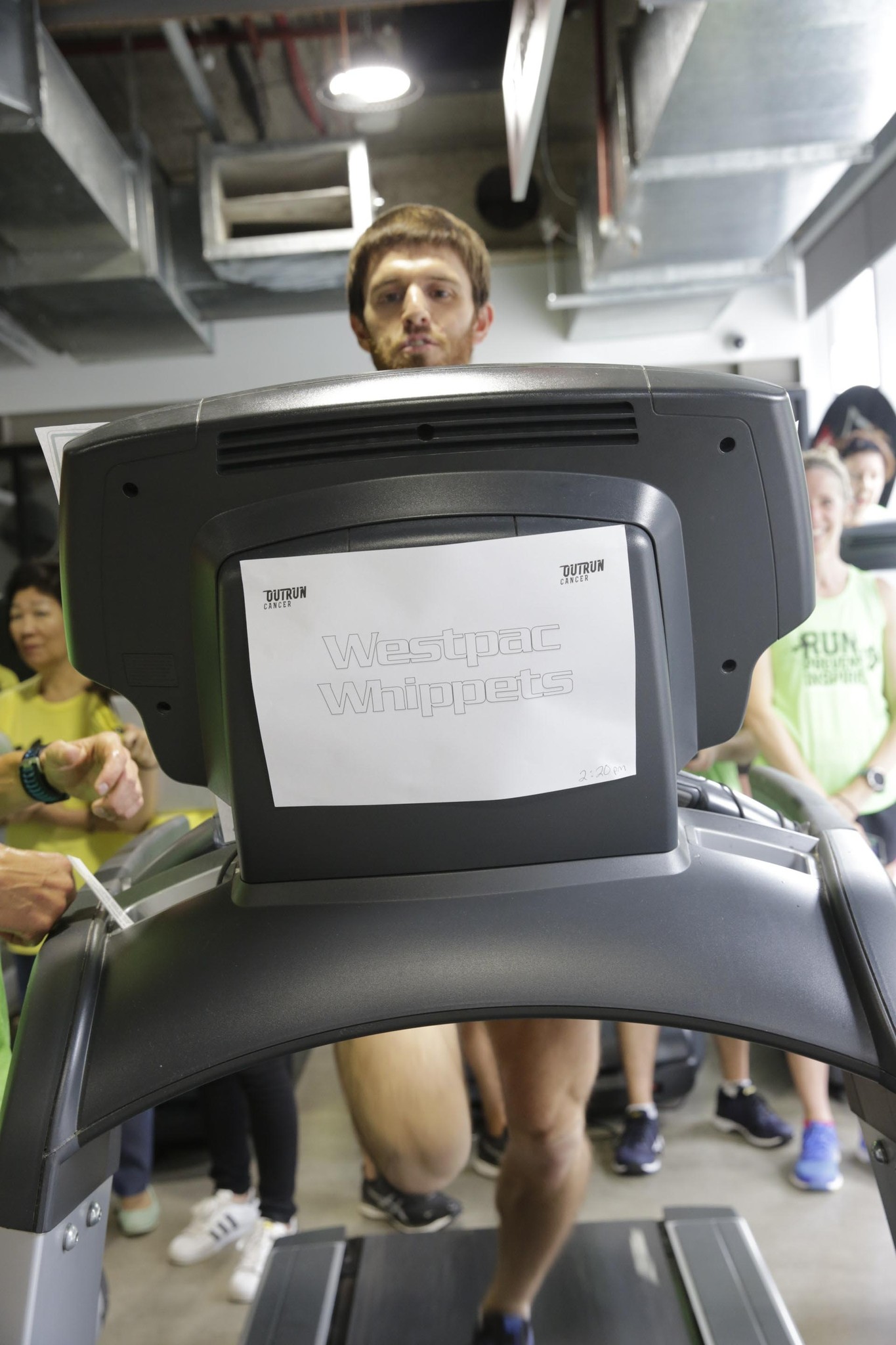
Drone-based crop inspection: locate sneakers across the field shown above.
[168,1189,261,1264]
[359,1173,462,1232]
[473,1130,510,1176]
[229,1219,300,1301]
[612,1110,666,1175]
[790,1124,841,1191]
[714,1083,792,1147]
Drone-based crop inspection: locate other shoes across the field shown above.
[117,1184,163,1237]
[471,1307,535,1345]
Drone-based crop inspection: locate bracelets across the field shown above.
[20,739,69,805]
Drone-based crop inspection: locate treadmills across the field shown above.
[0,356,895,1345]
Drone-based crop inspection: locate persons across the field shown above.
[329,202,604,1345]
[0,434,896,1301]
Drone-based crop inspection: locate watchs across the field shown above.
[859,768,887,798]
